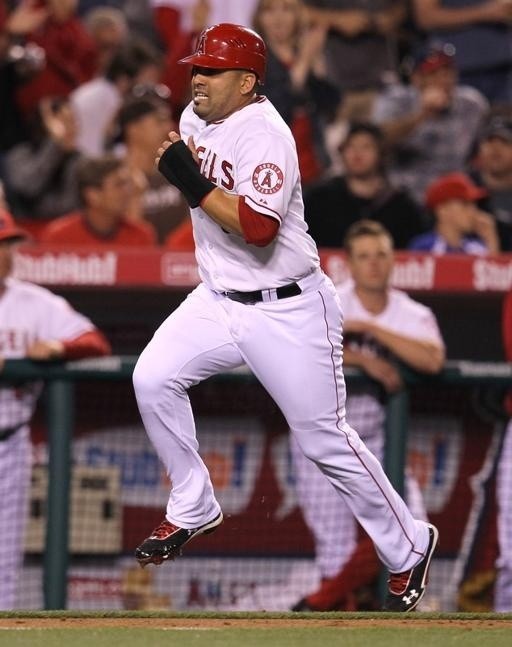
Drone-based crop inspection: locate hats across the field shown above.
[0,212,25,241]
[426,175,488,206]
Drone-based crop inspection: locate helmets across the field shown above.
[177,22,268,81]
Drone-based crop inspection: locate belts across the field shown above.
[220,284,300,304]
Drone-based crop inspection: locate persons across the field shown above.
[297,216,447,606]
[1,215,114,608]
[130,20,440,611]
[491,284,512,608]
[1,1,512,255]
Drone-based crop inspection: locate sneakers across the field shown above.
[381,523,439,612]
[134,509,223,570]
